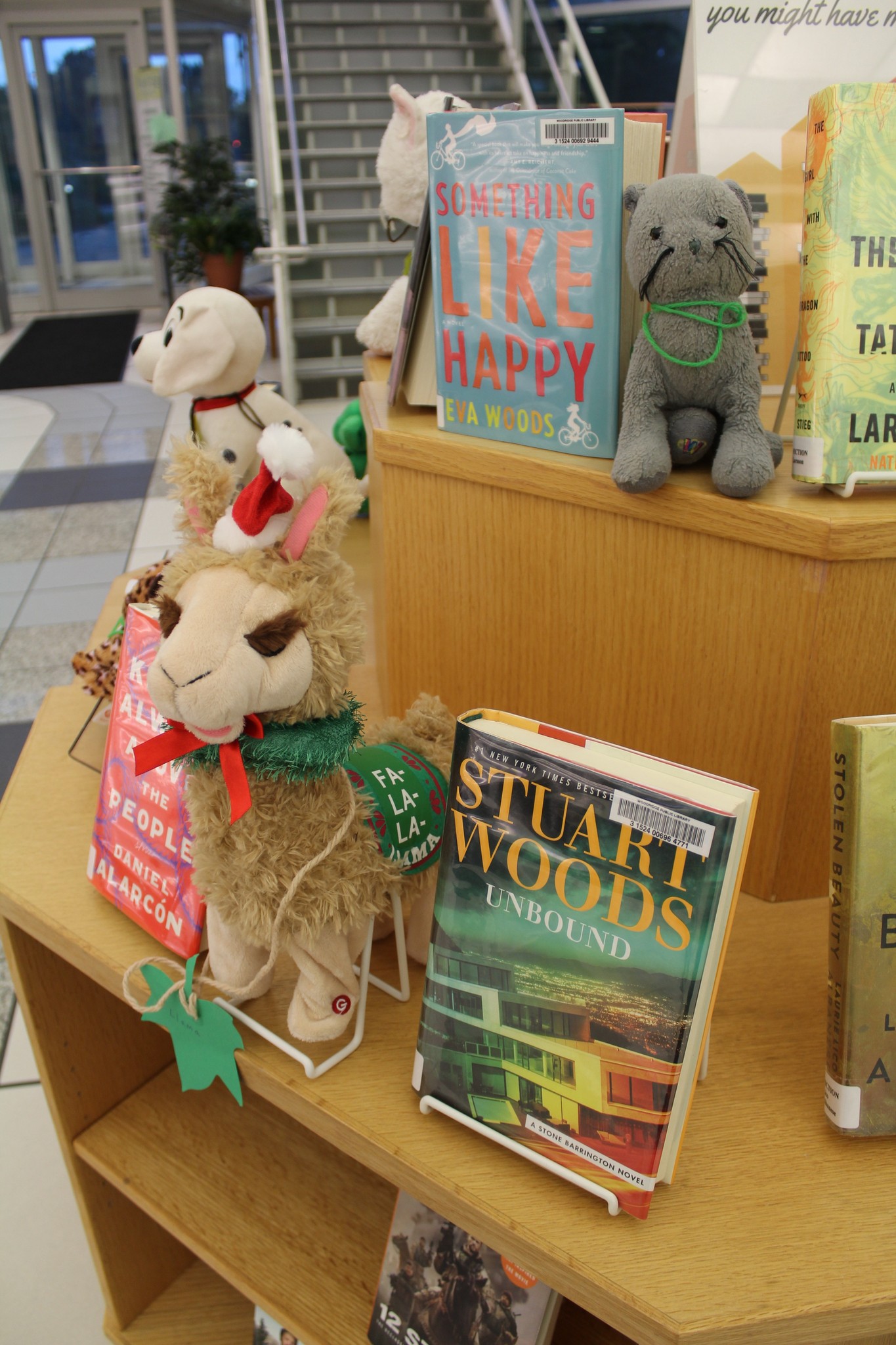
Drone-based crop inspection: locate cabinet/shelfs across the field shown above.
[1,556,896,1345]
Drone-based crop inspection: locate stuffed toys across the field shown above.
[605,169,789,503]
[131,284,378,523]
[134,420,456,1047]
[349,78,479,359]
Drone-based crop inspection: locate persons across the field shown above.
[280,1328,300,1345]
[385,1221,522,1345]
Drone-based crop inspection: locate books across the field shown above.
[822,711,896,1139]
[366,1188,565,1345]
[422,106,672,461]
[789,79,893,488]
[253,1306,304,1345]
[388,183,438,405]
[409,707,760,1222]
[81,598,222,961]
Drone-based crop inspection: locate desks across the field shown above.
[239,289,277,358]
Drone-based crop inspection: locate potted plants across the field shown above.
[147,136,270,293]
